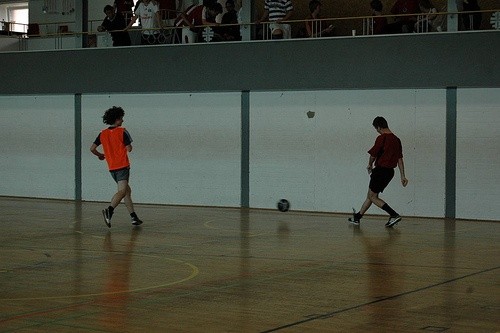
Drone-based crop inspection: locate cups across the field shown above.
[437,26,442,32]
[352,30,355,36]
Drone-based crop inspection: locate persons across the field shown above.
[369,0,389,34]
[97,5,134,45]
[255,0,295,39]
[392,0,423,33]
[462,0,482,32]
[303,0,333,38]
[348,116,408,227]
[221,0,243,41]
[171,3,224,46]
[113,0,135,27]
[421,2,439,19]
[124,0,165,46]
[89,106,144,227]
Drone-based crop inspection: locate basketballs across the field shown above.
[278,199,289,212]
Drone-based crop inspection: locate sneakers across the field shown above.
[132,218,144,225]
[385,214,402,227]
[348,216,360,225]
[102,208,111,227]
